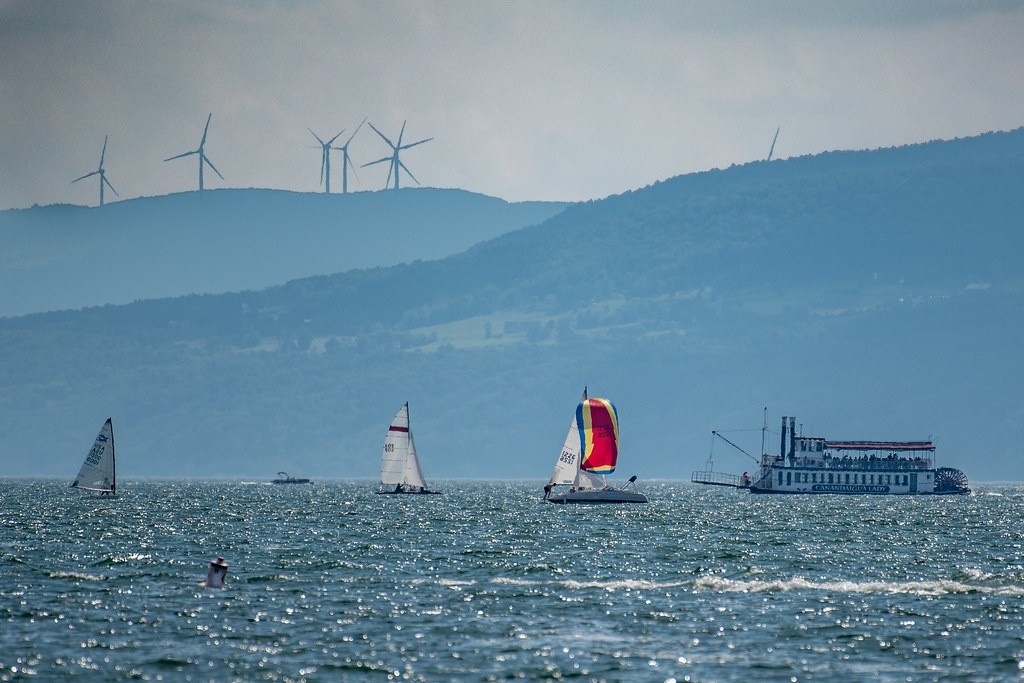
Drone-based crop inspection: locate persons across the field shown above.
[395,483,405,492]
[822,452,923,470]
[743,472,751,487]
[543,483,556,500]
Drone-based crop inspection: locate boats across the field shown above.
[746,415,972,494]
[270,472,313,484]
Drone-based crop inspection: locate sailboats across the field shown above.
[374,401,445,495]
[70,417,124,500]
[542,385,651,503]
